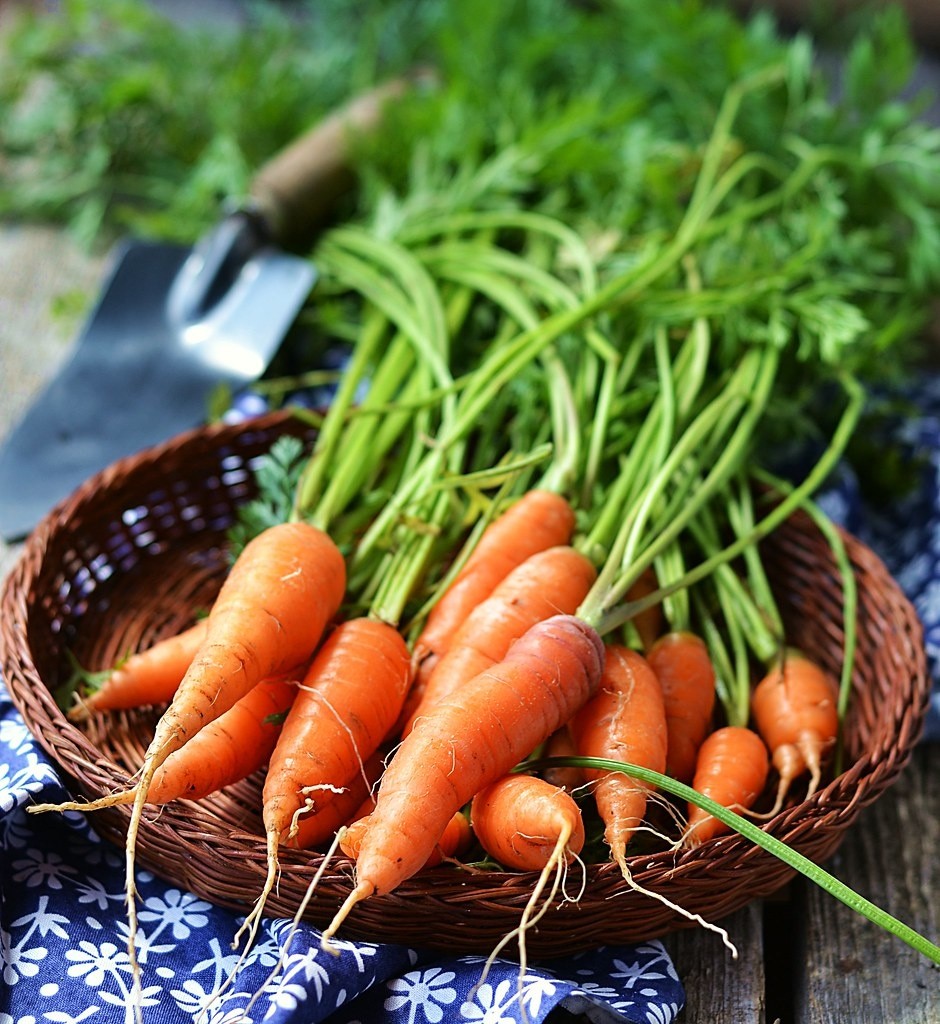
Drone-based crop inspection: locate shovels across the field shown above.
[1,61,442,542]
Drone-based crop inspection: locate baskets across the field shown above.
[0,400,931,961]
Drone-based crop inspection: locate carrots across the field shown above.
[17,490,841,1011]
